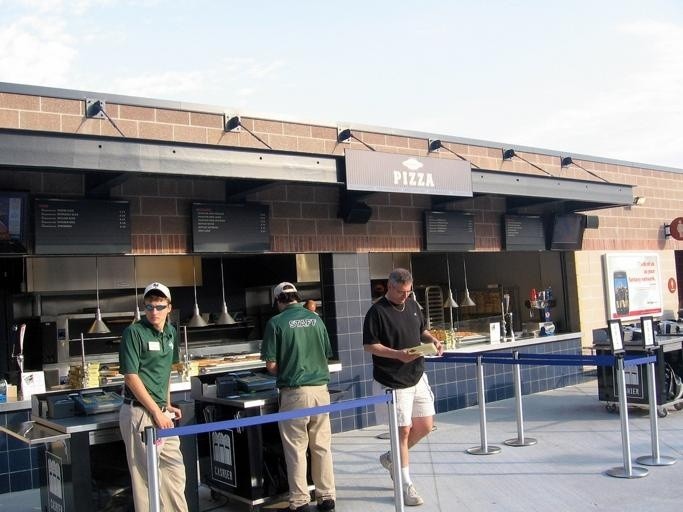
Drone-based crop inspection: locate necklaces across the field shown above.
[384,294,406,312]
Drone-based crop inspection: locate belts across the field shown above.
[122,398,167,414]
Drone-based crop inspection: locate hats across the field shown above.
[142,282,171,301]
[273,282,298,297]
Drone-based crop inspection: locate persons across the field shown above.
[266,282,335,508]
[118,283,189,507]
[362,268,443,504]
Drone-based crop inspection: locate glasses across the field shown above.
[143,303,168,311]
[393,285,414,295]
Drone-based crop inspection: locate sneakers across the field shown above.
[398,490,423,506]
[380,451,393,480]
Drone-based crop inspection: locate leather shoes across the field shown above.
[282,503,311,511]
[318,499,336,511]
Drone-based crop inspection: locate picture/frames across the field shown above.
[602,252,664,321]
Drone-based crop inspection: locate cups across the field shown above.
[0,381,7,404]
[490,322,500,346]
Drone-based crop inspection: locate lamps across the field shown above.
[215,256,237,325]
[459,255,476,307]
[128,255,141,327]
[87,256,111,334]
[186,256,209,328]
[443,253,460,308]
